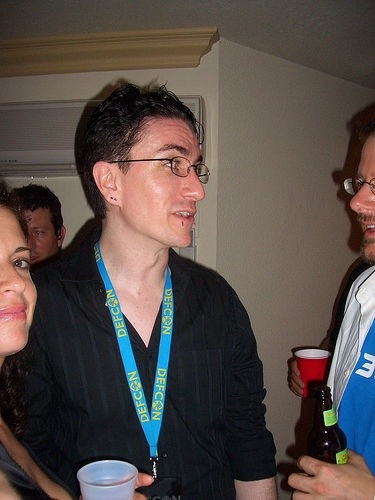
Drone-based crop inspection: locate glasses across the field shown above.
[344,177,375,196]
[111,156,211,185]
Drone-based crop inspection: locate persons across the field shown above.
[0,186,155,500]
[11,183,67,266]
[28,83,278,500]
[287,124,375,500]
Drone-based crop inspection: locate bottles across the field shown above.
[308,386,348,465]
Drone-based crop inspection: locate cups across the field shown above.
[294,349,330,397]
[77,460,138,500]
